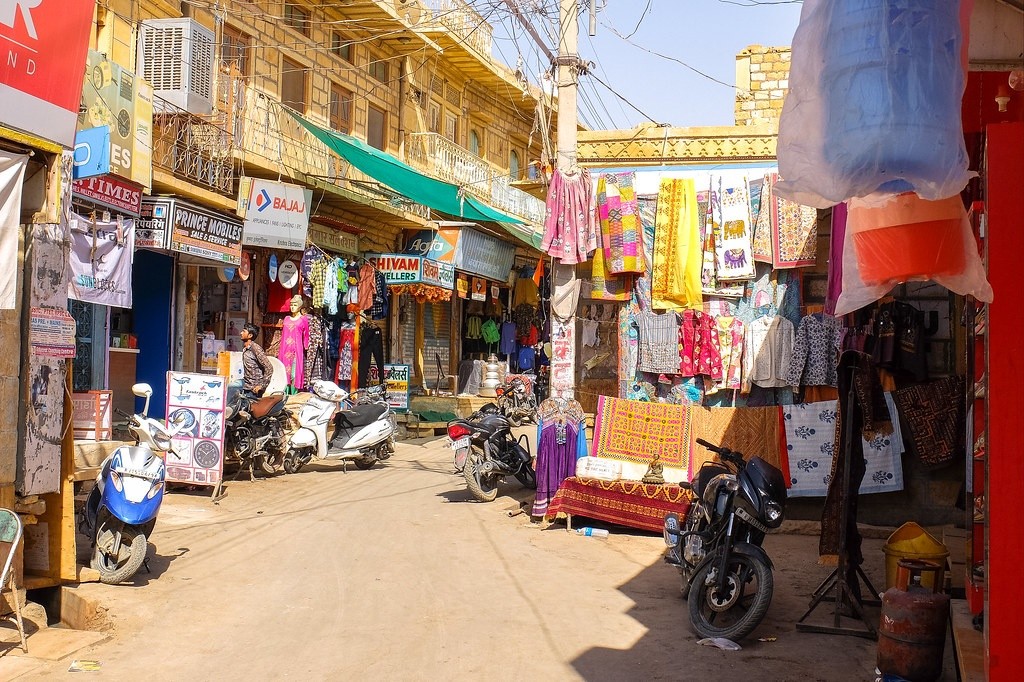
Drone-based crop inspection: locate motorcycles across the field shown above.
[223,390,289,483]
[496,369,539,427]
[447,385,537,502]
[663,438,788,642]
[78,383,186,586]
[284,380,395,474]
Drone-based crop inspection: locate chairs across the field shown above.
[0,508,28,654]
[435,353,457,396]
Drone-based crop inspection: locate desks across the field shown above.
[540,476,692,533]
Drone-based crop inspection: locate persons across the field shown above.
[281,294,309,394]
[241,323,273,398]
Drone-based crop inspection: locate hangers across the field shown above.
[554,388,569,425]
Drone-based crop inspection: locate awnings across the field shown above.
[270,98,527,224]
[496,222,548,255]
